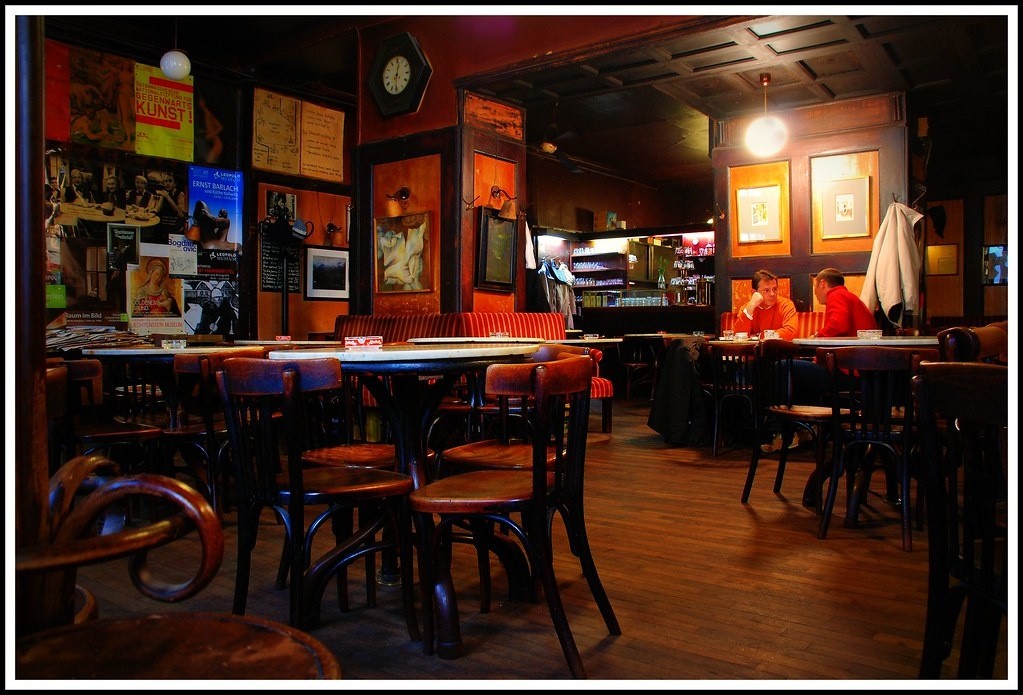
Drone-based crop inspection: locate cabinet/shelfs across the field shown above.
[570,251,625,289]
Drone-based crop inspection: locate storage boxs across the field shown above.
[593,209,618,231]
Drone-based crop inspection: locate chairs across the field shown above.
[17,337,1006,681]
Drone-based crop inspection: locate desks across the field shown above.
[793,336,940,526]
[233,340,342,449]
[80,345,265,496]
[267,344,539,645]
[407,337,545,444]
[544,338,624,448]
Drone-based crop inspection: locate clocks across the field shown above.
[367,30,432,121]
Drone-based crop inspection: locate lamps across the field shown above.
[175,215,202,244]
[744,73,788,159]
[491,186,518,220]
[160,15,191,80]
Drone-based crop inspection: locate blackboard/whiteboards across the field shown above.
[260,233,302,294]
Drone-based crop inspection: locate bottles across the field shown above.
[573,262,599,270]
[573,278,595,286]
[698,274,706,304]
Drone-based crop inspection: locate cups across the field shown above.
[764,330,775,339]
[616,297,668,306]
[723,330,734,339]
[736,333,747,338]
[596,279,623,285]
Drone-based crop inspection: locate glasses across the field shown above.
[757,288,779,294]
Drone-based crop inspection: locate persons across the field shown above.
[69,47,136,145]
[50,169,185,243]
[729,270,799,386]
[759,267,879,454]
[198,99,223,163]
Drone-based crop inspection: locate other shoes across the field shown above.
[761,432,798,453]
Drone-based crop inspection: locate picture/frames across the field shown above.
[474,206,520,293]
[924,242,959,276]
[303,244,350,302]
[735,180,785,244]
[818,175,871,240]
[374,209,435,295]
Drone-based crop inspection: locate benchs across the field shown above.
[460,312,613,436]
[721,312,885,378]
[333,313,459,408]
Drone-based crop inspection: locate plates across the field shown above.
[734,339,751,341]
[760,339,782,343]
[719,337,732,341]
